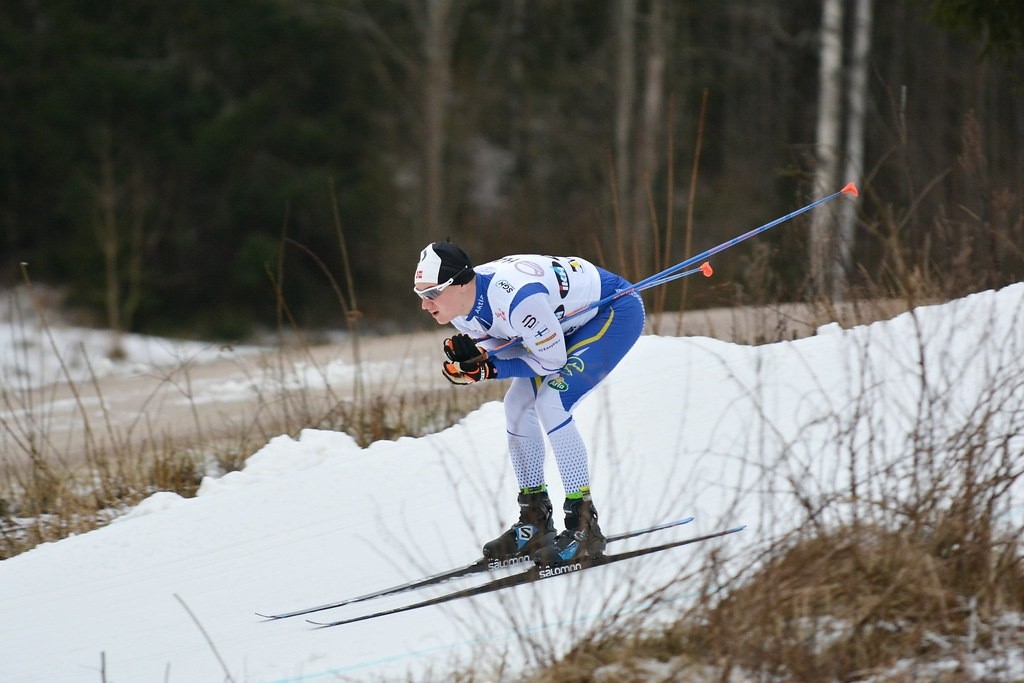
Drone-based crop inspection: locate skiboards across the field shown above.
[253,515,748,628]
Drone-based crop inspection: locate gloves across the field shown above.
[443,333,483,362]
[442,361,497,385]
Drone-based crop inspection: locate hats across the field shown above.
[414,241,475,286]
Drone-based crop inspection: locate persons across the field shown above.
[414,240,645,565]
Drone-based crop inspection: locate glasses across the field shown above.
[414,265,468,300]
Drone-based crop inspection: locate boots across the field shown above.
[483,492,558,560]
[534,498,607,566]
